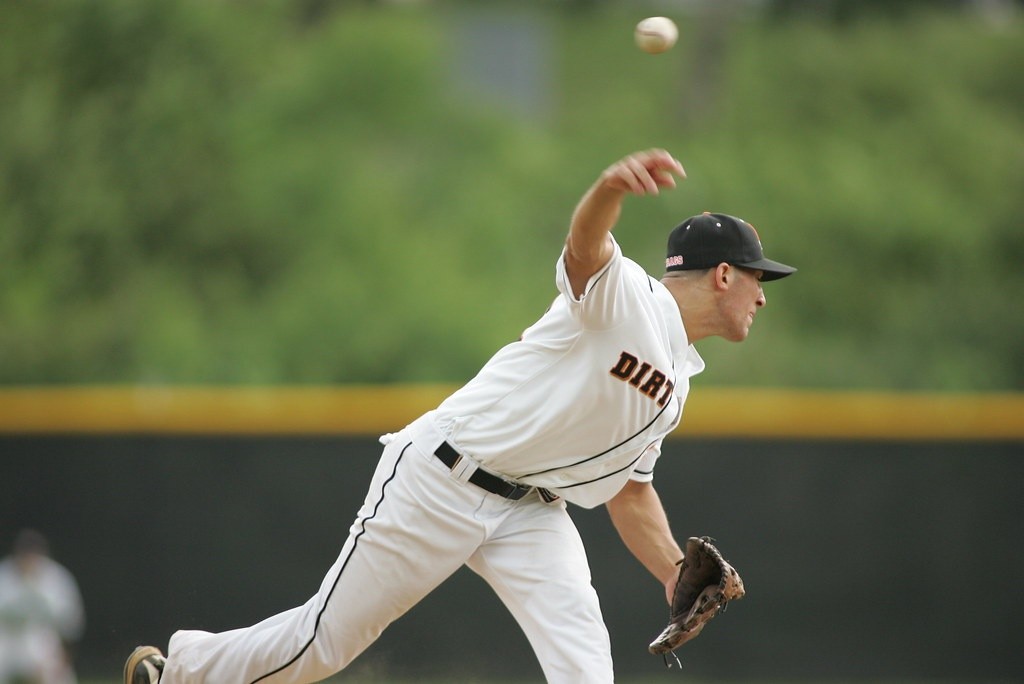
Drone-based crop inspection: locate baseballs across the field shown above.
[630,16,682,54]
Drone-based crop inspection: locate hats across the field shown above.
[665,212,798,282]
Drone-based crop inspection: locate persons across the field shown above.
[122,148,767,684]
[0,526,87,684]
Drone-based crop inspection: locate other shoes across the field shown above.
[123,646,167,684]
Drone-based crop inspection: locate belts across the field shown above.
[435,442,532,500]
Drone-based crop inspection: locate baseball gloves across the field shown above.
[644,533,750,669]
